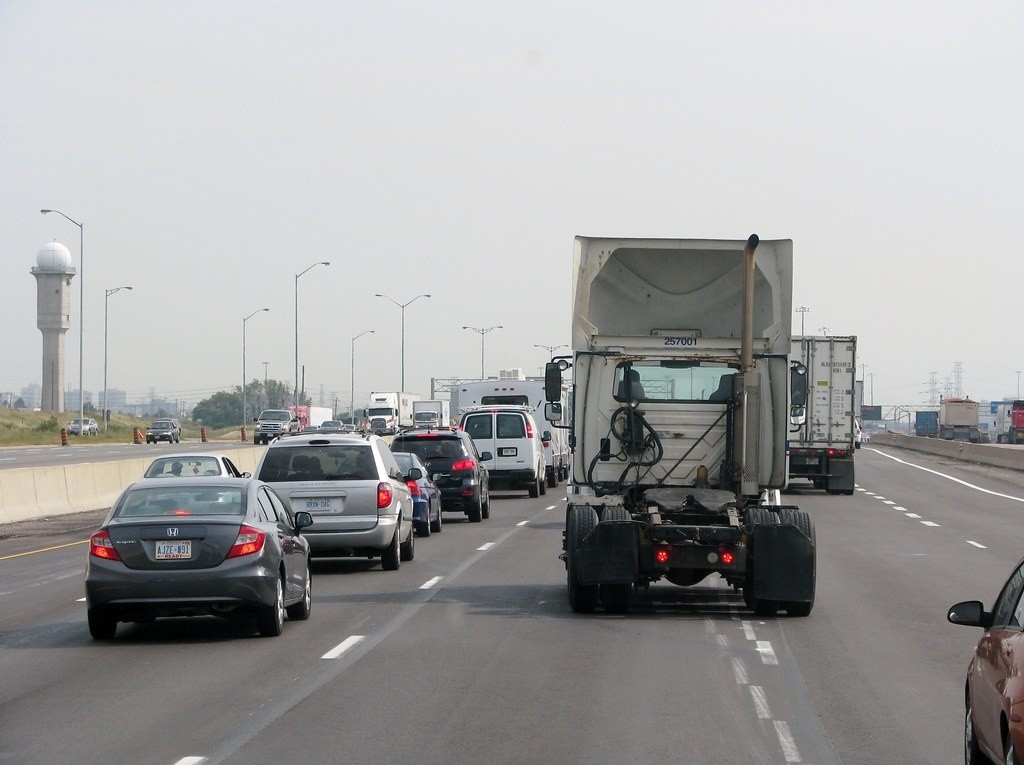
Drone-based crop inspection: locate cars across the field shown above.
[85,405,546,641]
[947,556,1024,765]
[980,432,991,443]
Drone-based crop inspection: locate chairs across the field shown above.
[708,374,736,402]
[620,370,649,398]
[148,498,177,513]
[288,455,312,480]
[137,504,161,516]
[208,503,244,516]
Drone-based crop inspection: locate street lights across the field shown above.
[243,309,270,432]
[859,363,867,405]
[352,331,375,424]
[104,286,133,433]
[41,209,84,438]
[1016,371,1022,400]
[375,294,431,393]
[463,326,504,379]
[868,372,878,406]
[534,344,569,363]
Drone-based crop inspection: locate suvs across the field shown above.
[67,416,98,436]
[855,420,861,449]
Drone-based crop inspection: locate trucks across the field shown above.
[915,411,937,438]
[938,398,979,442]
[993,399,1024,444]
[459,380,571,486]
[364,392,421,435]
[410,400,450,429]
[782,335,857,495]
[543,236,817,617]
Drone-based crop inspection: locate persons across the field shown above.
[172,462,183,476]
[351,452,378,479]
[623,369,640,383]
[289,416,299,431]
[308,456,325,475]
